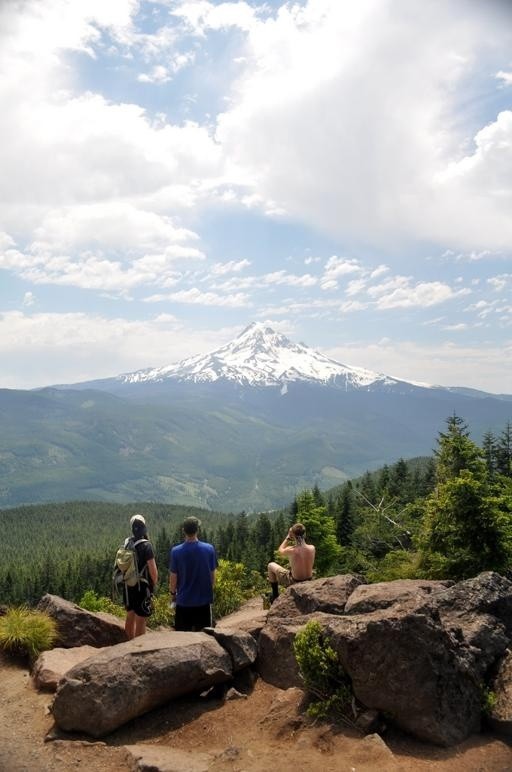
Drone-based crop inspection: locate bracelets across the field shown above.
[287,537,290,541]
[170,592,176,595]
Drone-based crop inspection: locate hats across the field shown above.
[184,516,201,528]
[130,515,145,527]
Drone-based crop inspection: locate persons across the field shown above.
[122,515,159,639]
[169,516,218,631]
[267,523,317,603]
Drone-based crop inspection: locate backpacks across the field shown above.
[112,537,149,587]
